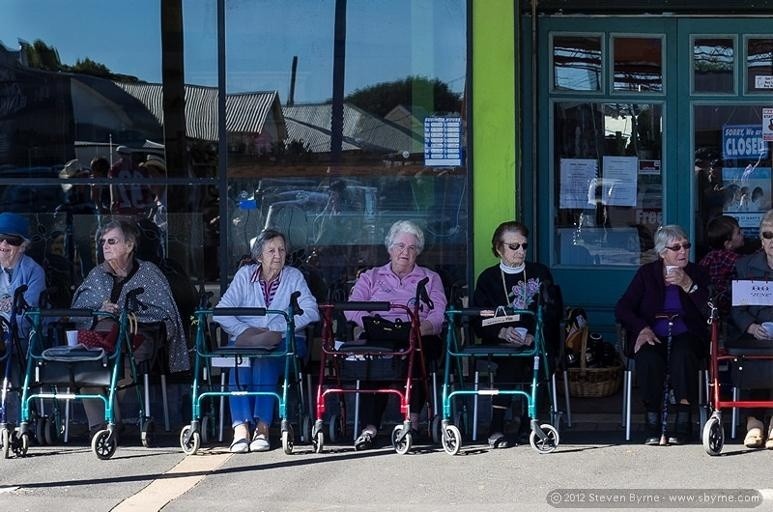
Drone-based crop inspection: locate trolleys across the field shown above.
[306,275,444,458]
[434,278,566,455]
[8,290,159,462]
[178,280,312,456]
[0,283,53,456]
[694,285,772,460]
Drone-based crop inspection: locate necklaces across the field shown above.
[501,270,527,310]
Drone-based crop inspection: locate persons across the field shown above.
[314,181,359,291]
[564,126,589,157]
[471,223,564,448]
[697,216,747,319]
[695,145,771,224]
[725,210,773,449]
[70,218,190,439]
[614,225,716,446]
[343,218,448,450]
[51,141,224,283]
[0,211,52,371]
[213,228,320,454]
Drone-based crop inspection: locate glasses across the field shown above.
[504,243,528,250]
[763,232,773,239]
[665,242,691,251]
[0,234,24,246]
[99,239,118,245]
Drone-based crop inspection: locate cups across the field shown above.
[761,322,773,340]
[666,265,679,276]
[515,327,527,343]
[65,331,77,346]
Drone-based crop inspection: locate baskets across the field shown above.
[556,326,625,398]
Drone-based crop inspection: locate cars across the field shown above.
[204,177,378,239]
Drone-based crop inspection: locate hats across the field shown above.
[139,155,167,172]
[0,212,29,239]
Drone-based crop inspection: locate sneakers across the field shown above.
[487,439,509,447]
[249,439,271,451]
[744,432,763,447]
[230,438,249,452]
[354,433,379,450]
[765,438,773,449]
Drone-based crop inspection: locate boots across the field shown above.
[643,402,661,445]
[668,404,691,444]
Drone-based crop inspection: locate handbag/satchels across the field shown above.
[237,328,282,346]
[361,316,412,349]
[78,324,146,355]
